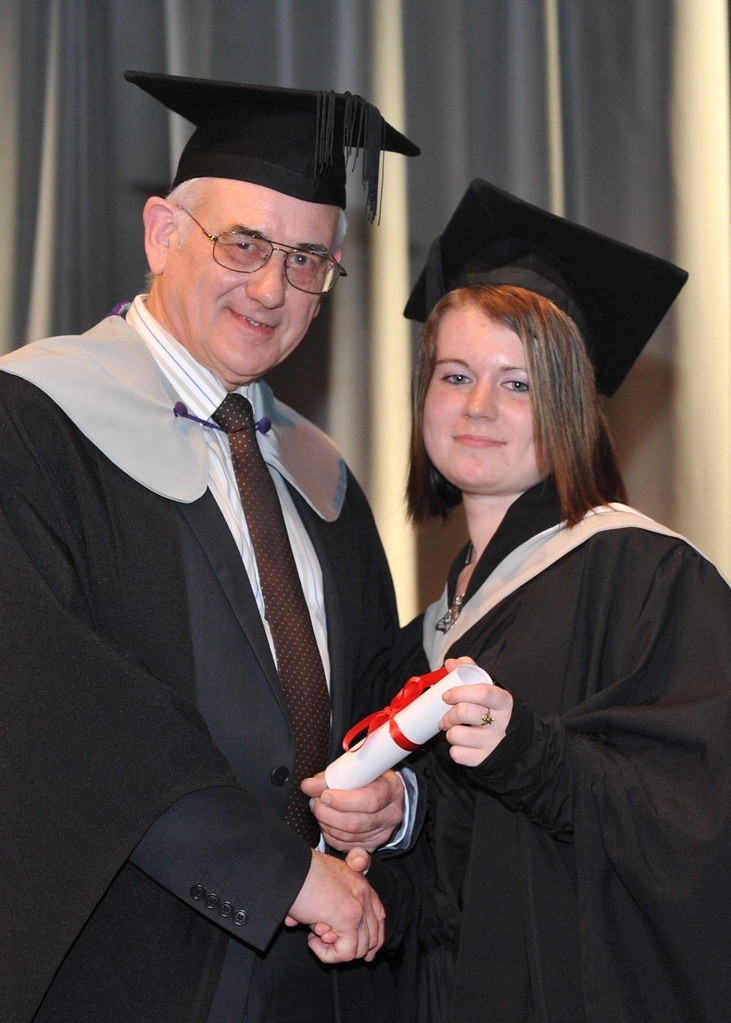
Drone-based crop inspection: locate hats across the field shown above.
[123,65,421,229]
[407,175,688,400]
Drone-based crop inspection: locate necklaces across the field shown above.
[434,542,472,632]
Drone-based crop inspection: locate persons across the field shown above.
[0,69,423,1023]
[285,178,731,1023]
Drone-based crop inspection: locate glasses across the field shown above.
[175,202,347,294]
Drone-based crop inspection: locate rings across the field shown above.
[480,707,494,726]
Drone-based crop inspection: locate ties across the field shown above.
[211,393,331,854]
